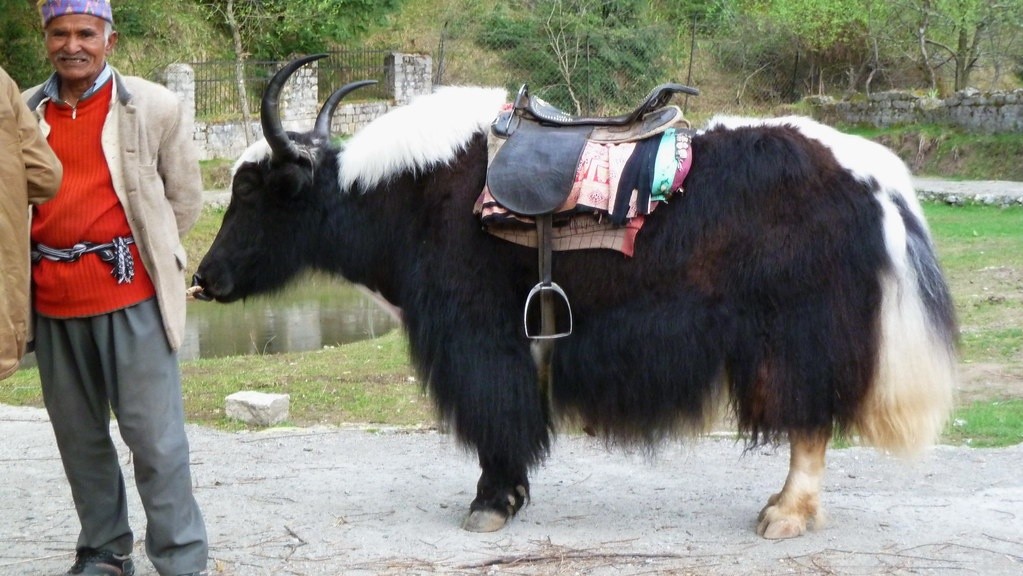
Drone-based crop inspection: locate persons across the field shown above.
[0,65,64,382]
[20,0,209,576]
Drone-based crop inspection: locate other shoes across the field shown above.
[65,548,135,576]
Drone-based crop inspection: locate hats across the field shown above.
[37,0,113,29]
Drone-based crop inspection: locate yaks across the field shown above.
[191,53,962,541]
